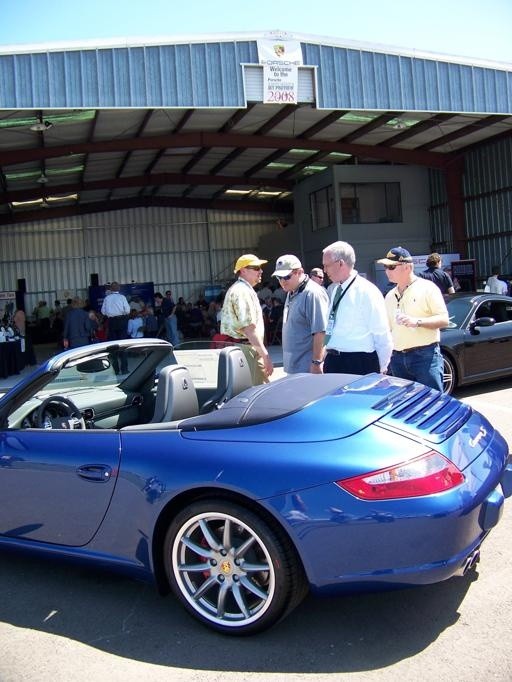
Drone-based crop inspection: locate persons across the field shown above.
[270,255,331,375]
[221,254,273,385]
[14,299,98,367]
[100,268,326,344]
[378,247,449,391]
[419,254,508,296]
[323,242,394,375]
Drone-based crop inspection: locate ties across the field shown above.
[324,285,343,346]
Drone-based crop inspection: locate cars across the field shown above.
[440,292,512,397]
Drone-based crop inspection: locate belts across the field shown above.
[332,349,345,356]
[401,343,436,354]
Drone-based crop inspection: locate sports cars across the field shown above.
[1,337,511,612]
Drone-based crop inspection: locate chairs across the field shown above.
[148,345,252,424]
[269,315,284,345]
[132,326,149,338]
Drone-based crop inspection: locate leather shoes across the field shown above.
[122,370,130,374]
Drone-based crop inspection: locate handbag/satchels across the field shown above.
[211,334,240,348]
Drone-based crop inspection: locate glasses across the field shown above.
[384,264,401,271]
[247,266,262,271]
[275,271,295,281]
[317,275,324,280]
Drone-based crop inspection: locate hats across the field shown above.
[235,254,269,271]
[377,247,413,266]
[426,253,441,267]
[271,254,302,277]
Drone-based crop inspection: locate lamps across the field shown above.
[36,168,49,183]
[30,112,53,131]
[393,117,406,129]
